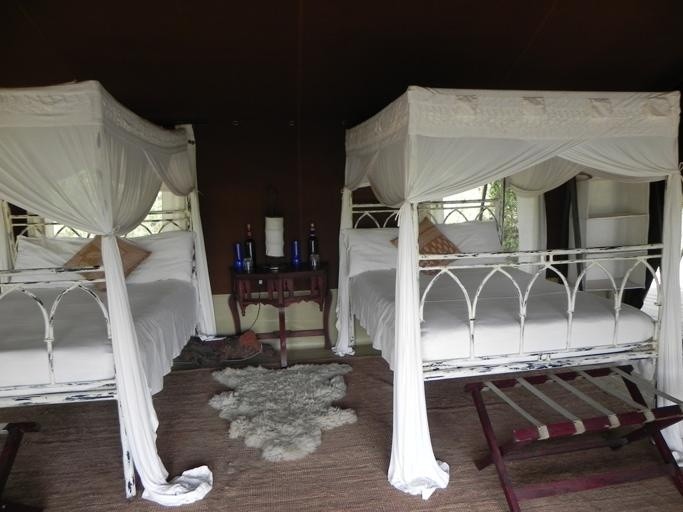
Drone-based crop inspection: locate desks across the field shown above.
[224,262,336,369]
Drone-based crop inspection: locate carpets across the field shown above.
[204,364,359,461]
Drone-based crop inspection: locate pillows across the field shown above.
[54,232,151,289]
[388,217,464,273]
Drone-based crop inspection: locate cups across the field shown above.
[308,253,320,270]
[242,257,254,273]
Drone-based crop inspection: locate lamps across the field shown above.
[261,216,287,271]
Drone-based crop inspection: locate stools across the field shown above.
[457,366,683,510]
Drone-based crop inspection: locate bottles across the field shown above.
[305,223,320,266]
[243,223,256,267]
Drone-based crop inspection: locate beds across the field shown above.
[0,206,218,504]
[336,202,683,449]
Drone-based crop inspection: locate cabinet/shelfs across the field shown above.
[577,173,651,292]
